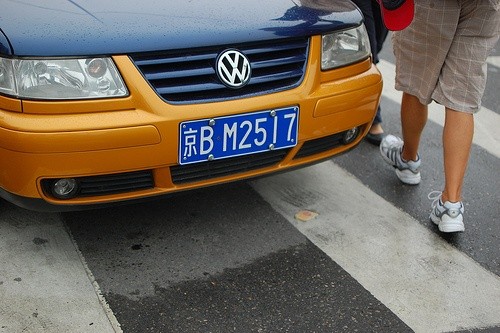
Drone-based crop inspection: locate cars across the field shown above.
[0,0,383,213]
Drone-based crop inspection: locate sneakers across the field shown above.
[427,191,465,233]
[380,135,422,185]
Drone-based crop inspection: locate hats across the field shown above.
[377,0,415,31]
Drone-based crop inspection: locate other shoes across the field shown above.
[365,132,385,145]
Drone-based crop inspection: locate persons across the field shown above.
[380,0,500,233]
[351,0,390,146]
